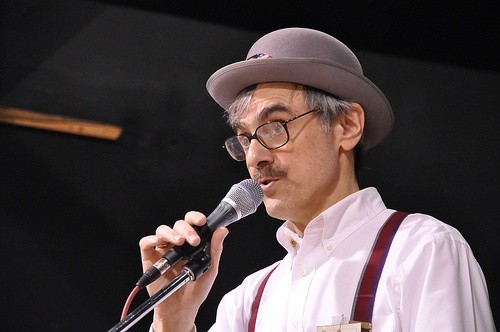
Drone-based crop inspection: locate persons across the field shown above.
[133,25,499,332]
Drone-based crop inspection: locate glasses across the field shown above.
[224,108,314,161]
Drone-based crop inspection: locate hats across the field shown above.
[206,27,395,151]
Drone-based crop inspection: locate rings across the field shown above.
[156,243,169,248]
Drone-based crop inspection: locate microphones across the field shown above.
[136,178,264,290]
